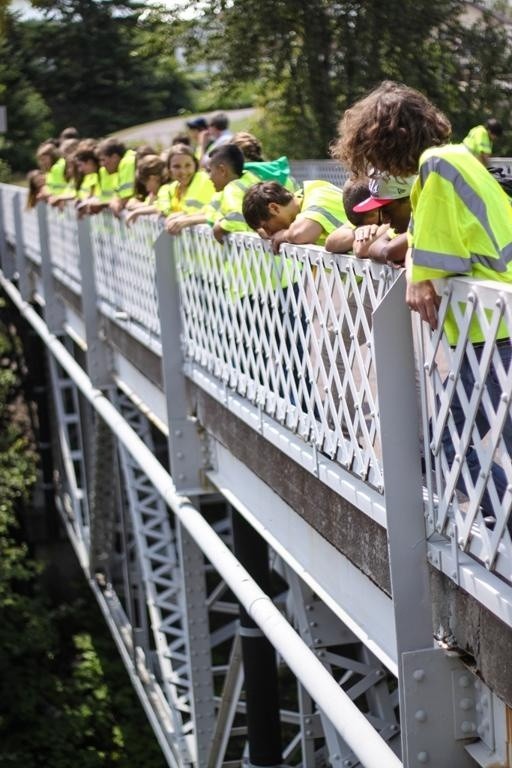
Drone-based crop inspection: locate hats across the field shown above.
[352,167,419,213]
[186,118,207,129]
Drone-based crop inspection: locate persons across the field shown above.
[21,110,298,246]
[321,171,392,255]
[241,177,355,256]
[347,163,421,262]
[457,117,505,172]
[326,78,512,545]
